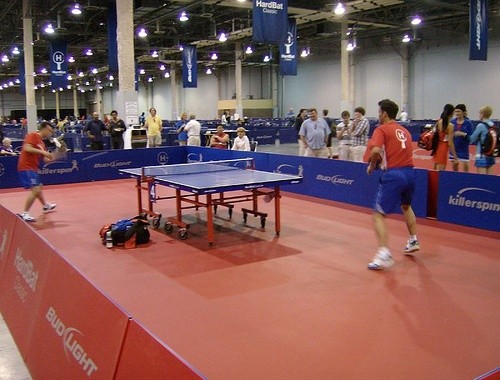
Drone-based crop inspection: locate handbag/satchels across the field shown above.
[100,215,150,246]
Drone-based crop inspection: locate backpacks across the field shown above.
[478,122,500,157]
[418,123,438,151]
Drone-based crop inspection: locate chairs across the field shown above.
[204,133,260,152]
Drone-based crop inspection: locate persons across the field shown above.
[184,114,200,146]
[287,108,295,118]
[144,108,162,147]
[470,105,495,174]
[295,109,307,155]
[176,112,189,146]
[399,111,408,122]
[210,126,229,149]
[299,109,331,158]
[231,127,250,151]
[347,107,369,161]
[363,99,420,269]
[139,112,145,126]
[432,105,459,171]
[336,111,353,160]
[18,122,57,222]
[322,109,333,158]
[222,111,247,129]
[448,104,474,172]
[0,110,127,156]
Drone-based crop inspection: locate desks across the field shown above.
[118,157,304,249]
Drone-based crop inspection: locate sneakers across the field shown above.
[22,213,34,221]
[368,253,395,270]
[401,239,420,253]
[44,203,57,212]
